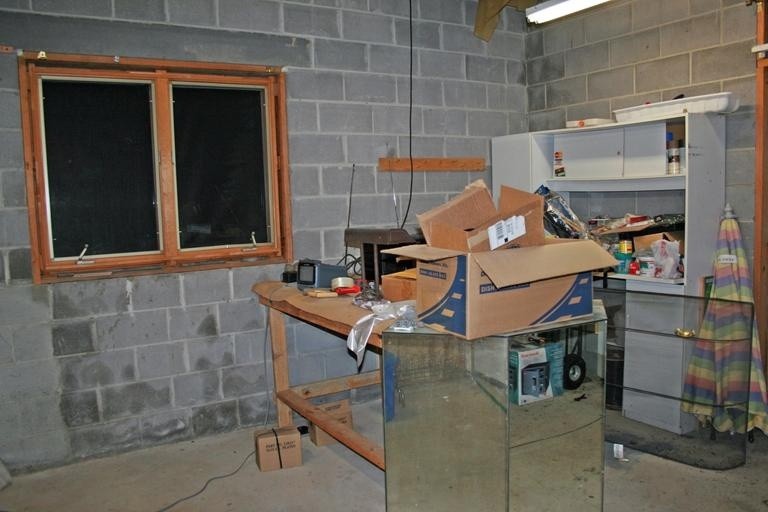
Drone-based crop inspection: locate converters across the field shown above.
[283,270,297,283]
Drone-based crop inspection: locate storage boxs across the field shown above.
[380,240,621,341]
[255,427,302,472]
[415,184,543,253]
[308,398,352,448]
[510,341,564,405]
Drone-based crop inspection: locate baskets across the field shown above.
[566,118,615,128]
[613,92,733,123]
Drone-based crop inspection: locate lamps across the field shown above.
[526,0,614,25]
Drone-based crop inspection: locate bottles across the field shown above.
[553,151,565,178]
[667,140,681,176]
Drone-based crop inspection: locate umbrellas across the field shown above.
[681,202,767,442]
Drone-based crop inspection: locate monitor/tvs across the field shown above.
[297,259,346,290]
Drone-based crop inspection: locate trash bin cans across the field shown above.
[605,338,624,411]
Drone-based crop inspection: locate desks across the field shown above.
[253,281,398,471]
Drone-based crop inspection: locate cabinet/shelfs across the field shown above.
[467,112,727,435]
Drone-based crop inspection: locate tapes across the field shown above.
[331,276,355,291]
